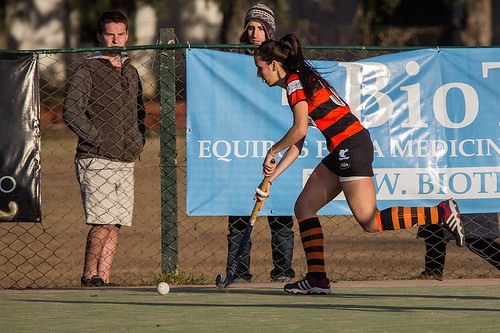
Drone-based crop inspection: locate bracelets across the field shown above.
[268,179,273,186]
[271,145,275,156]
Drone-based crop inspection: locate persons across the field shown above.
[61,9,146,288]
[253,33,464,295]
[216,4,296,283]
[413,213,500,281]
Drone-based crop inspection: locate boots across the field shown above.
[268,228,296,283]
[224,229,253,283]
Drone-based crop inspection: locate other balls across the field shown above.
[157,282,170,296]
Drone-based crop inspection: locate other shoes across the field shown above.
[103,281,120,287]
[80,275,104,287]
[413,270,444,281]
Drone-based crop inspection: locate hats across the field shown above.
[238,2,276,44]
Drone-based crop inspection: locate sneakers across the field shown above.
[437,198,466,247]
[282,273,332,296]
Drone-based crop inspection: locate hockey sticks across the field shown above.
[214,159,276,294]
